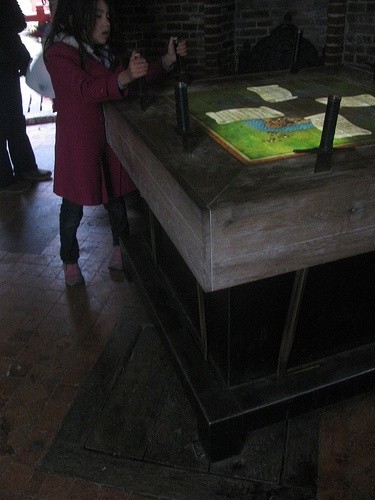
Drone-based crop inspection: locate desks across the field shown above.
[100,60,374,385]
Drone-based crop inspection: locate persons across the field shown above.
[0,0,51,196]
[40,0,188,292]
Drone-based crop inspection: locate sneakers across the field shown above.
[63,263,85,286]
[107,245,124,271]
